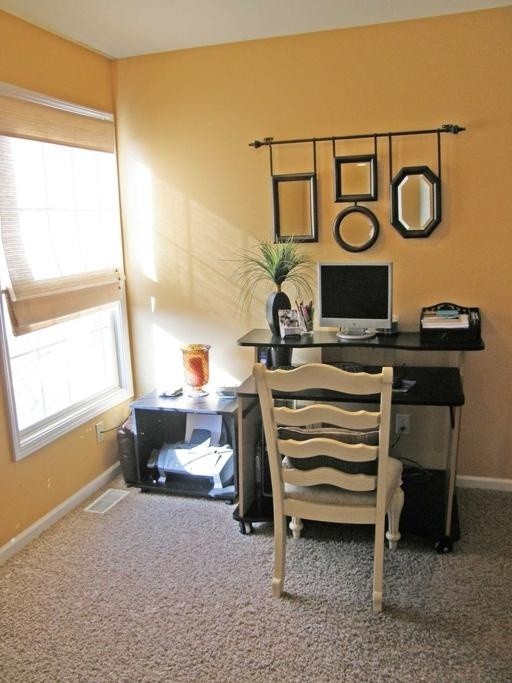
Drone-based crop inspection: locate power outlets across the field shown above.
[96,421,105,444]
[395,414,410,434]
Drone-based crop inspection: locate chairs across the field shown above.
[253,362,404,611]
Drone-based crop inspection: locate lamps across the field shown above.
[179,343,211,397]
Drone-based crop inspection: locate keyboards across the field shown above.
[278,362,366,376]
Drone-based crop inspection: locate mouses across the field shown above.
[393,376,403,389]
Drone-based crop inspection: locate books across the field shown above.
[421,310,469,329]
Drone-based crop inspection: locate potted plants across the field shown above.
[217,232,317,336]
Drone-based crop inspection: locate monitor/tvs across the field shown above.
[317,262,393,340]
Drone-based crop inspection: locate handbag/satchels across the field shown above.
[401,468,461,542]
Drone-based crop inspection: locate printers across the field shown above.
[146,414,235,491]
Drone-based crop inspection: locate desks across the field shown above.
[128,389,239,505]
[234,330,485,554]
